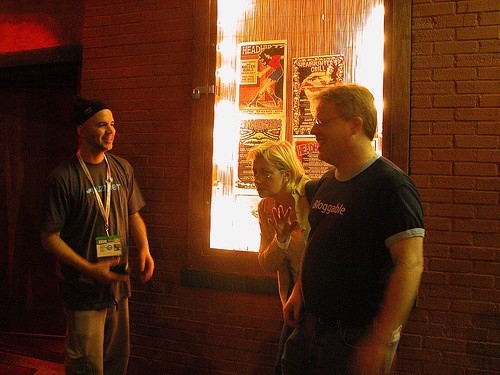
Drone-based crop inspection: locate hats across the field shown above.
[72,96,112,127]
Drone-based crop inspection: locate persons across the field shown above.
[249,141,311,375]
[40,100,154,375]
[116,245,119,250]
[282,84,425,375]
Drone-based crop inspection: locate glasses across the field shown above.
[252,169,288,179]
[313,116,340,126]
